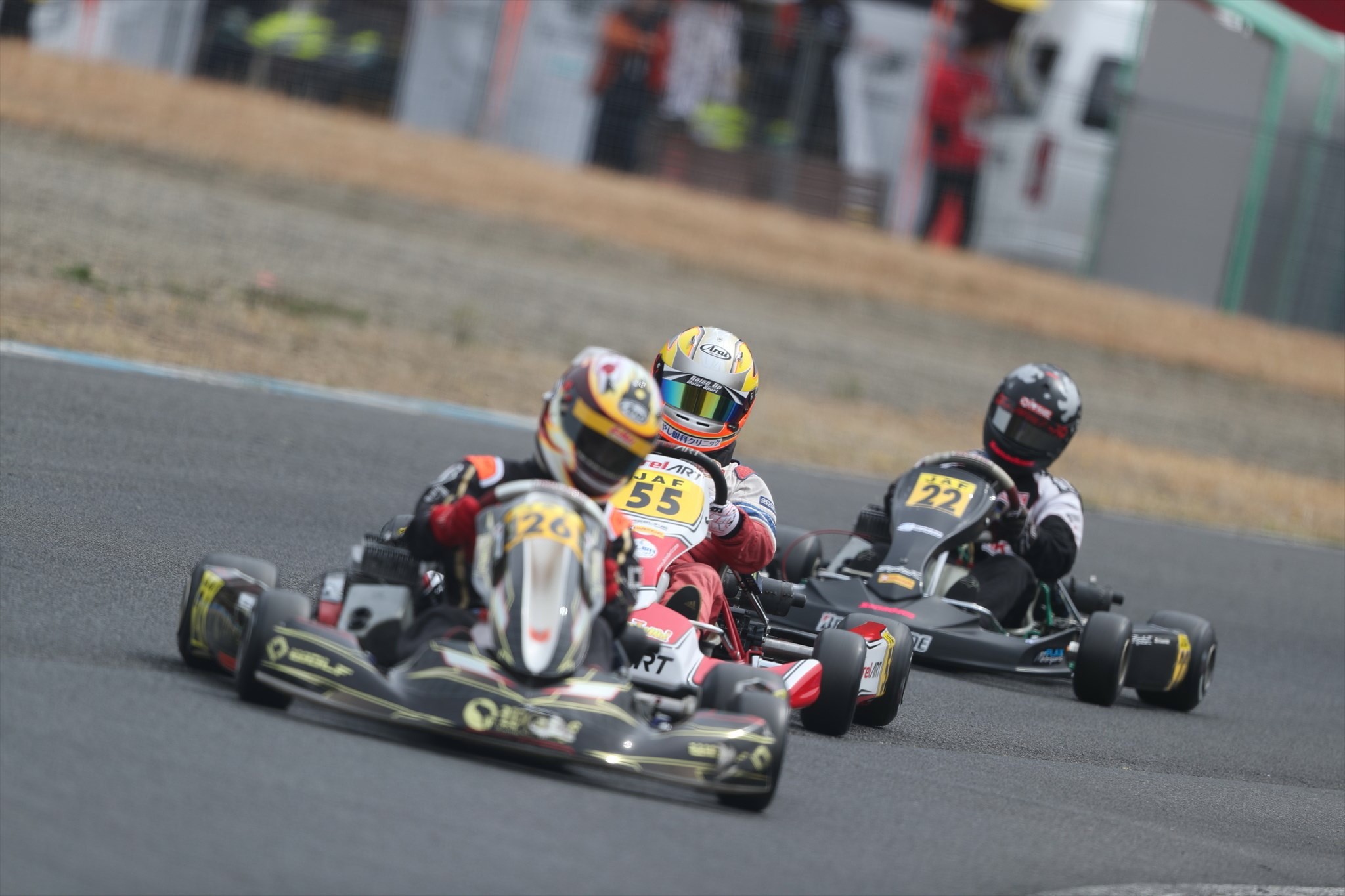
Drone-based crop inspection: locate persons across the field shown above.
[589,0,673,172]
[412,346,664,680]
[837,361,1084,629]
[649,323,778,620]
[733,0,852,202]
[913,29,998,251]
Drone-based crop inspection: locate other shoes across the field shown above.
[663,584,703,621]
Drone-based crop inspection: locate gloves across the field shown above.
[707,499,739,536]
[431,495,480,547]
[1030,516,1077,581]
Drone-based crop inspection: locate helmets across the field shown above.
[533,346,664,507]
[652,326,759,452]
[984,362,1083,473]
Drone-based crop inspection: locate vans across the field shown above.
[963,0,1151,270]
